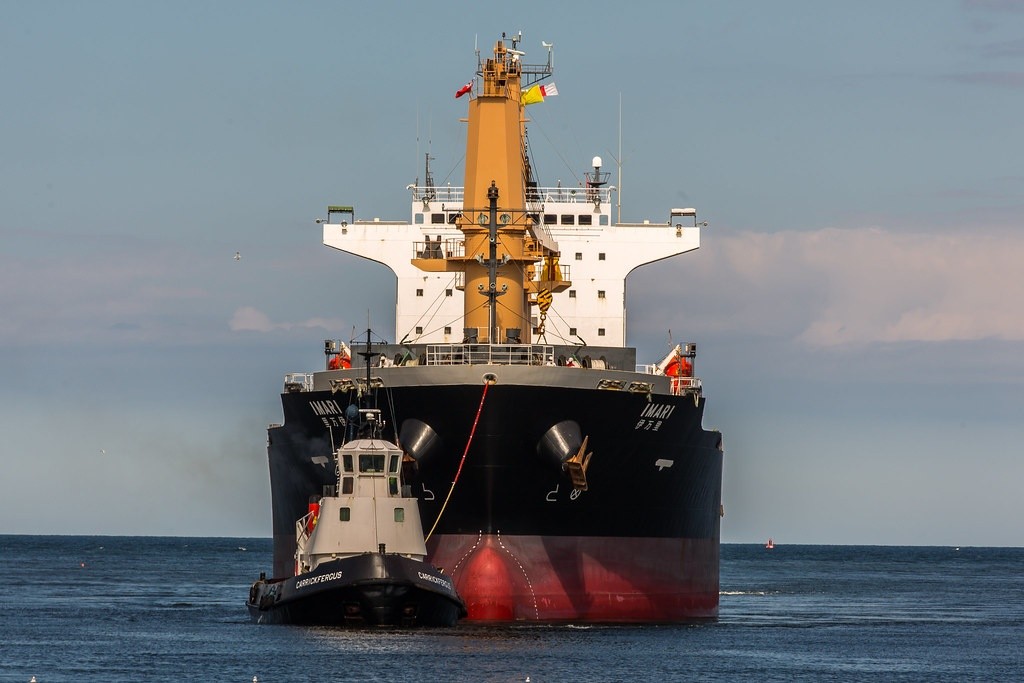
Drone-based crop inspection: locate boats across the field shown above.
[246,306,468,628]
[766,539,775,548]
[265,30,721,626]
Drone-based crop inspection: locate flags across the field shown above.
[455,82,471,99]
[539,85,546,97]
[521,84,543,106]
[544,82,558,96]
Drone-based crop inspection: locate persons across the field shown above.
[546,356,556,367]
[379,356,389,368]
[566,357,580,368]
